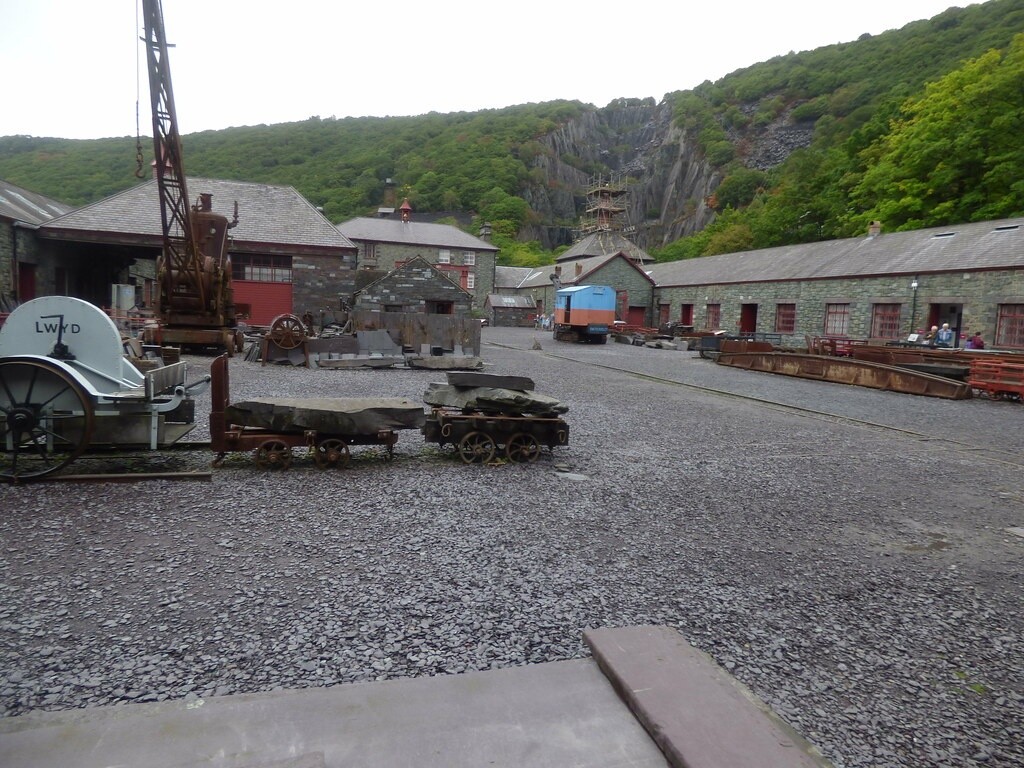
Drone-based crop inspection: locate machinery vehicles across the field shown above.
[133,0,246,356]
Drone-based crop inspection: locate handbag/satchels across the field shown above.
[921,340,930,345]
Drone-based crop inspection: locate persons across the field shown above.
[533,313,556,331]
[923,323,985,350]
